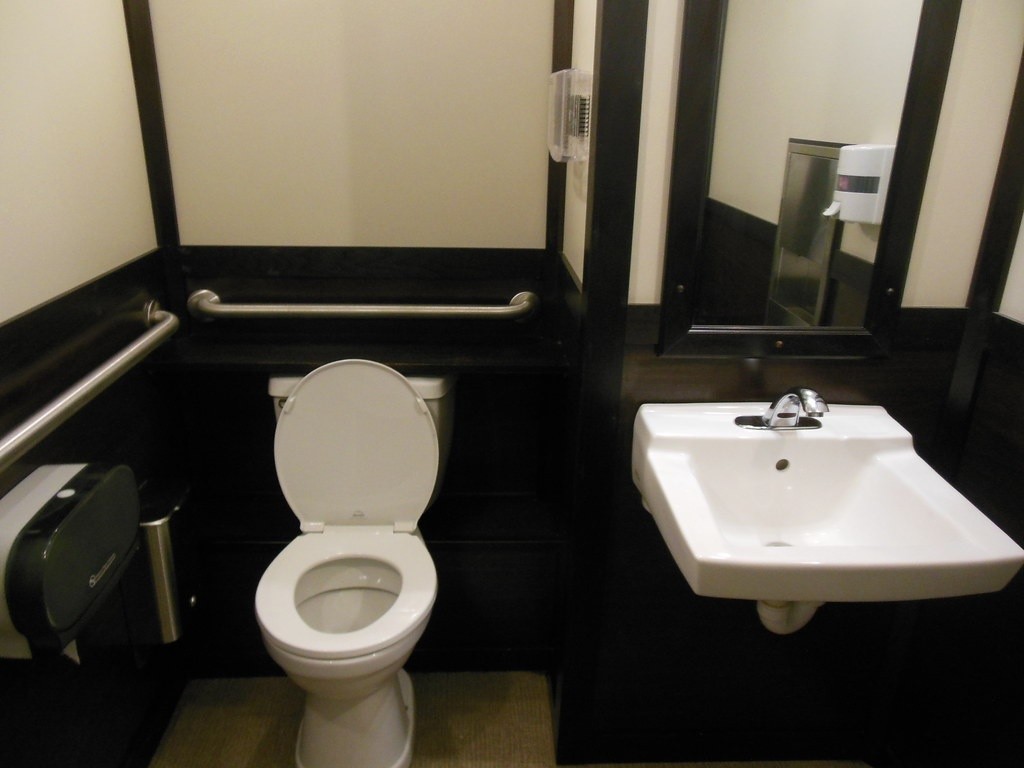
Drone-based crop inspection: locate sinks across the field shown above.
[632,401,1024,602]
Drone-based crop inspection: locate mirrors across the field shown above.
[654,0,962,362]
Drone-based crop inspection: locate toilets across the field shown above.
[254,357,451,765]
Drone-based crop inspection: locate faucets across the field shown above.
[764,386,830,430]
